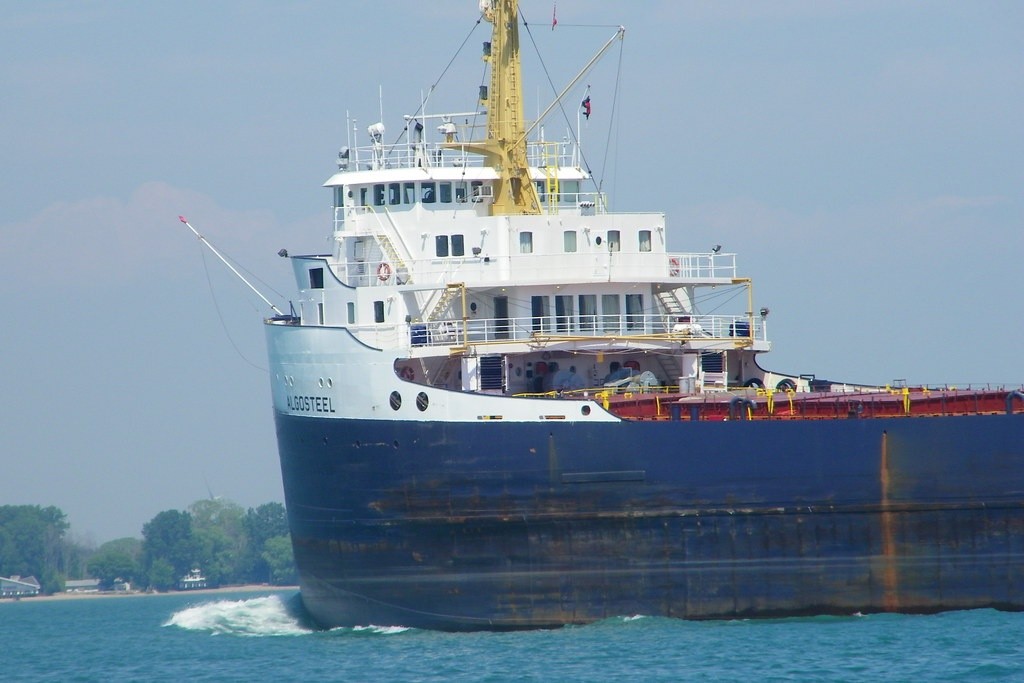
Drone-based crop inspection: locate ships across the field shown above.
[264,105,1023,618]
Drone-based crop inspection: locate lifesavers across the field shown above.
[376,262,390,281]
[400,366,415,381]
[669,257,680,276]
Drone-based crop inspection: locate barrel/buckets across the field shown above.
[688,377,695,393]
[679,377,689,394]
